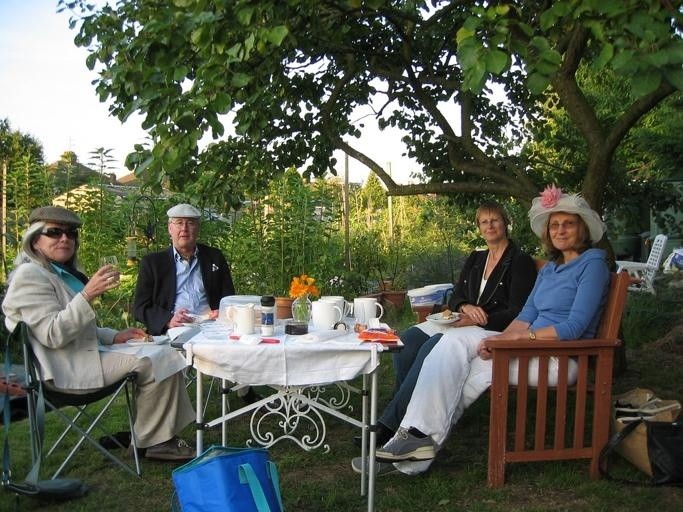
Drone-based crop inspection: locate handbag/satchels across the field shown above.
[597,387,683,486]
[430,288,455,314]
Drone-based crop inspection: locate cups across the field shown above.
[227,301,255,335]
[310,295,385,331]
[99,255,120,291]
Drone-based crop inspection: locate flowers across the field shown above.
[285,272,322,318]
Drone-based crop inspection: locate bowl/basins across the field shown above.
[200,321,233,338]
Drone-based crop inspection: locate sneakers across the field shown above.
[375,427,435,463]
[350,456,401,478]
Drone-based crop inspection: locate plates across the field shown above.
[423,310,463,324]
[124,334,167,348]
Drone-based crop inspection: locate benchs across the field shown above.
[414,270,632,490]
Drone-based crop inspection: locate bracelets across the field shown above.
[80,290,91,301]
[461,303,467,313]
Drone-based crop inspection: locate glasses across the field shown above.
[548,220,578,228]
[38,227,78,240]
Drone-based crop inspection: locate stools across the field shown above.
[172,348,233,417]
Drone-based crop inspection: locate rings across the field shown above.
[473,314,477,318]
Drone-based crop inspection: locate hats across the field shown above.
[166,204,201,219]
[526,182,607,245]
[28,205,83,227]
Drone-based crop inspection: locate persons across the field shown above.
[0,205,195,460]
[133,204,254,403]
[351,212,611,477]
[352,200,537,452]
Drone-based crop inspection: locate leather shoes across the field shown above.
[240,386,255,402]
[350,422,394,448]
[144,434,196,459]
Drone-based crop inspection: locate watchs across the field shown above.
[528,329,536,340]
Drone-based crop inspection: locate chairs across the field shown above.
[614,234,669,296]
[5,318,144,496]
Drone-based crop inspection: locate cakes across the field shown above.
[441,310,454,320]
[143,334,153,342]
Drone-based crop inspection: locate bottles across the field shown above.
[259,295,276,337]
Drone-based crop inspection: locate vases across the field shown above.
[352,280,433,324]
[290,294,313,328]
[273,297,294,306]
[273,306,292,320]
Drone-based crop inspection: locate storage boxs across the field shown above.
[406,282,454,312]
